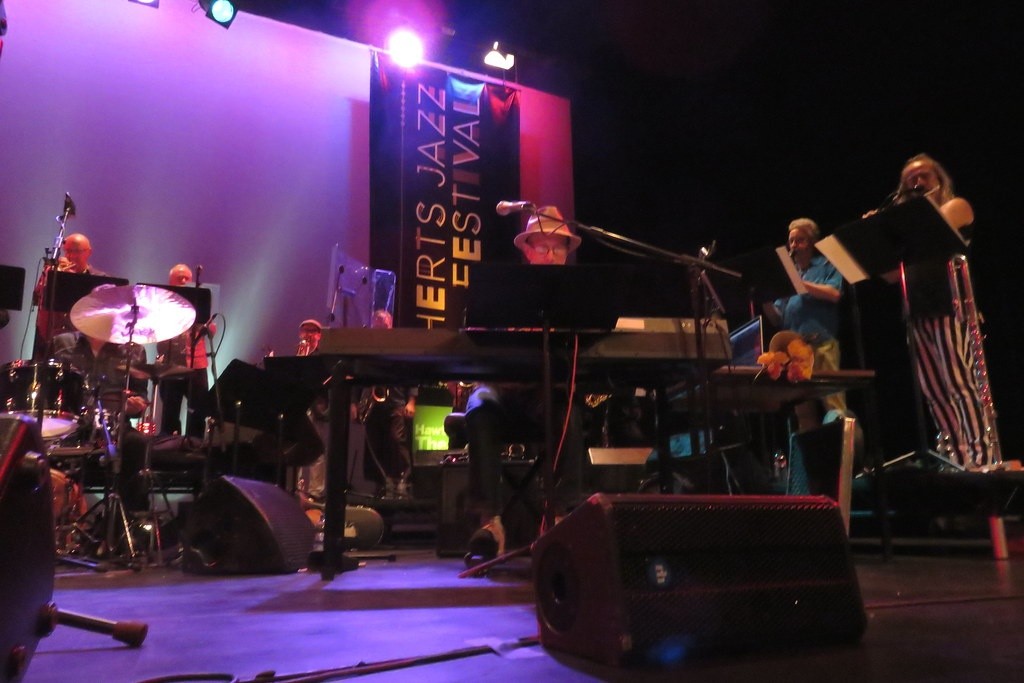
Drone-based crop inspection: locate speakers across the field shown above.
[208,359,325,466]
[436,463,537,551]
[182,475,316,577]
[530,492,867,667]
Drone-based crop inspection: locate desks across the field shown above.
[264,355,729,579]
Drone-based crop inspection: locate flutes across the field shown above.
[948,251,1009,473]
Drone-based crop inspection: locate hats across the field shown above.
[514,206,581,253]
[298,320,322,329]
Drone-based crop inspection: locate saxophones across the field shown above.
[296,335,312,356]
[353,383,390,426]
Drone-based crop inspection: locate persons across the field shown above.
[463,206,597,556]
[759,217,871,472]
[290,310,420,500]
[862,153,1003,474]
[32,233,217,442]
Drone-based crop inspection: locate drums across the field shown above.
[51,466,90,560]
[1,359,91,443]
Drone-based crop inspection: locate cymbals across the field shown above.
[70,285,197,344]
[119,359,194,377]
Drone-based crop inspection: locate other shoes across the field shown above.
[468,516,505,561]
[382,491,414,500]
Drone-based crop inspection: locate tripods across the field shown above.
[75,306,180,572]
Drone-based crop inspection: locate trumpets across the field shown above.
[46,259,77,279]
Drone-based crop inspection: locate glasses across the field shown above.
[62,246,90,254]
[301,327,319,334]
[526,241,569,256]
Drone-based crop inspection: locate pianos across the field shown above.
[317,318,733,570]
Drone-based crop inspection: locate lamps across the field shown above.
[198,0,239,29]
[482,39,518,83]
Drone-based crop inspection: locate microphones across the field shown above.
[496,200,533,216]
[64,192,76,216]
[914,184,924,192]
[195,314,217,345]
[788,248,798,259]
[88,378,102,408]
